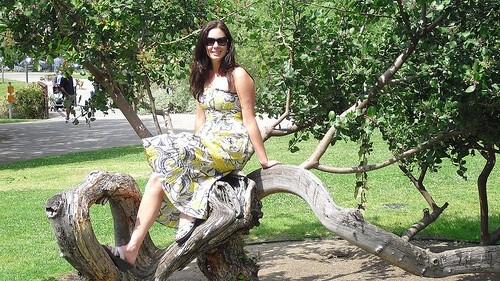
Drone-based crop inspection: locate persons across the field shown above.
[102,21,282,271]
[60,66,79,125]
[54,53,67,90]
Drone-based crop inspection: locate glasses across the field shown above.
[205,36,228,46]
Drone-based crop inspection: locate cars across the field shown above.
[0,56,83,71]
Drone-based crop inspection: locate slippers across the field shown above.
[175,215,204,243]
[101,243,134,273]
